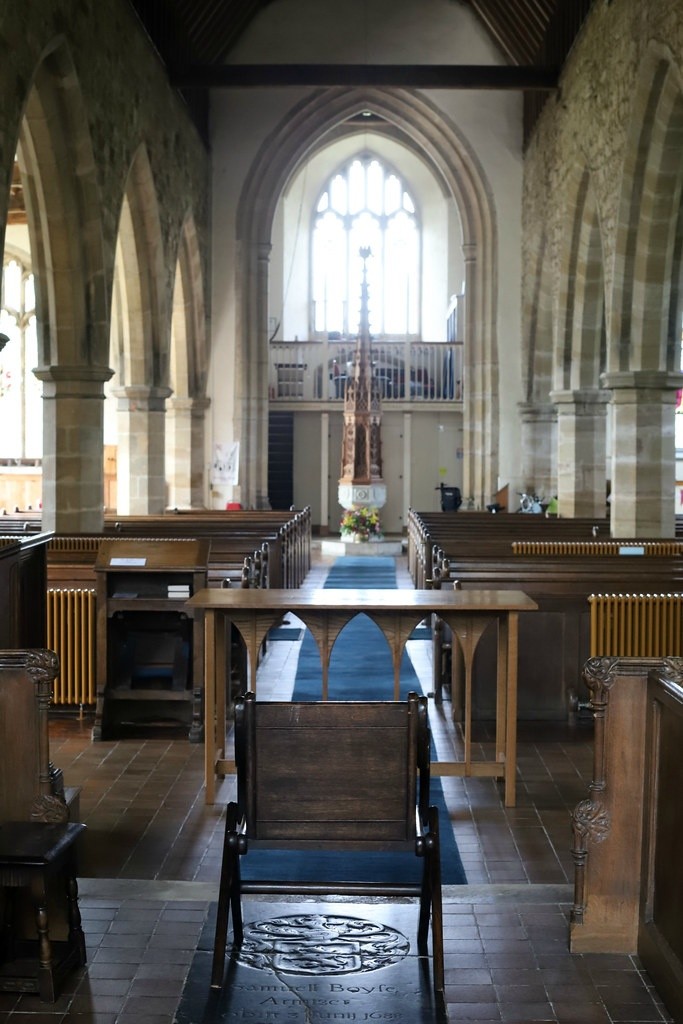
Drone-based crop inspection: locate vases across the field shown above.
[340,528,384,543]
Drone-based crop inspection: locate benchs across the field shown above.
[407,509,682,741]
[0,505,312,704]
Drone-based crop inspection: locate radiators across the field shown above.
[511,542,683,554]
[568,592,683,725]
[43,587,98,721]
[0,536,195,551]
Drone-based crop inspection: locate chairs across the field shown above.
[209,692,444,1009]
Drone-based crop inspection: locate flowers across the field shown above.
[340,508,382,542]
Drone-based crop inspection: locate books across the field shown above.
[167,584,192,598]
[112,592,138,598]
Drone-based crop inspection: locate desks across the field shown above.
[185,588,538,808]
